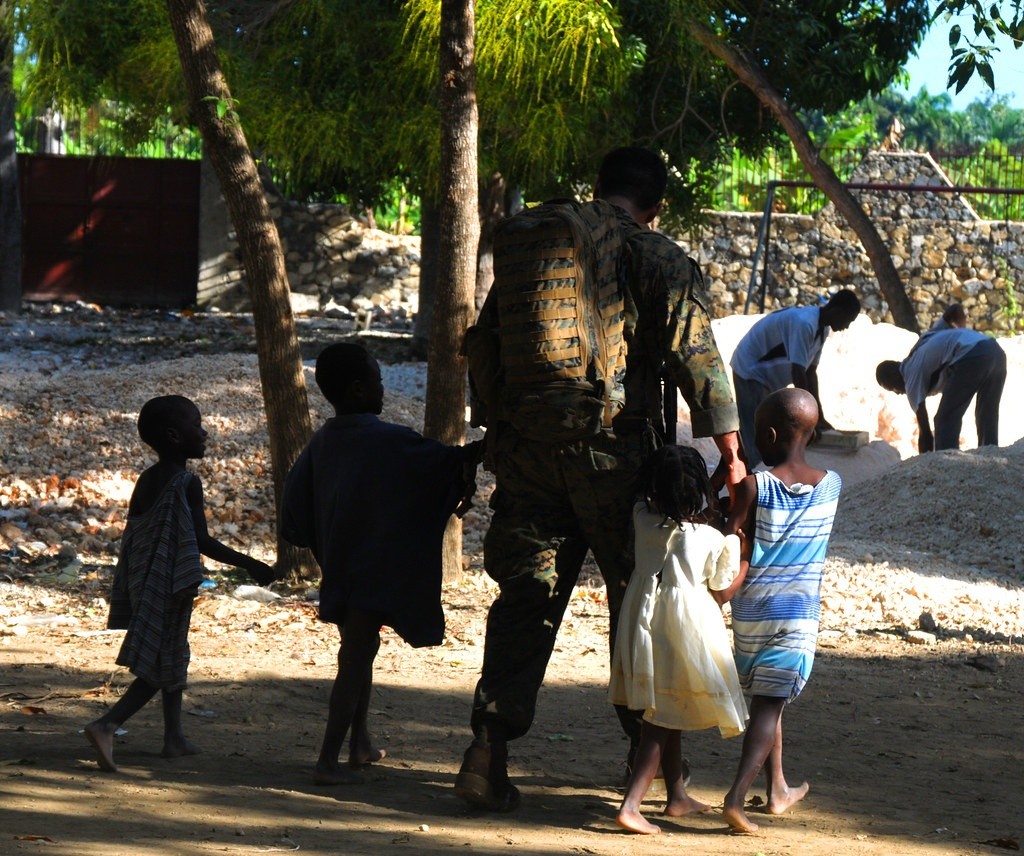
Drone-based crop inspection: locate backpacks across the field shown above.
[492,196,667,443]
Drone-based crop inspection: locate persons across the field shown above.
[607,445,753,836]
[711,388,842,830]
[281,343,487,782]
[730,288,1006,478]
[453,147,746,813]
[84,394,275,773]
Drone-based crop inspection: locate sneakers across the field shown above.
[626,749,690,795]
[456,741,520,813]
